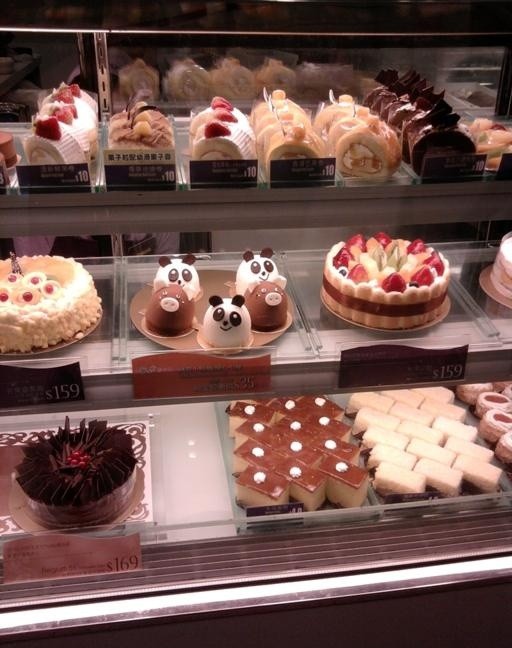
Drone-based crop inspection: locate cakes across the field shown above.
[0,252,101,352]
[490,237,512,299]
[15,416,137,529]
[322,231,450,329]
[145,247,288,352]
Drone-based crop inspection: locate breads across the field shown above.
[229,382,512,512]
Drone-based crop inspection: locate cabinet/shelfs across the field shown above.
[0,2,512,643]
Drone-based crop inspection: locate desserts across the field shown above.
[0,58,512,188]
